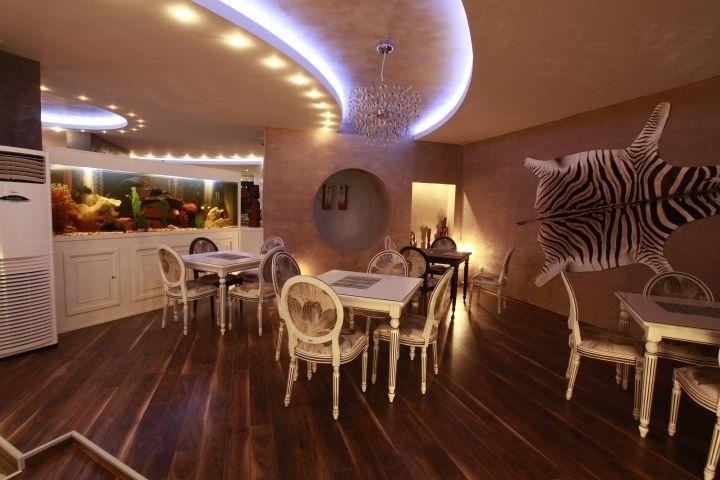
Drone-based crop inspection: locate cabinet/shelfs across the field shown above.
[52,229,240,335]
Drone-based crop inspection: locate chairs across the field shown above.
[397,246,440,292]
[667,366,719,480]
[227,248,287,335]
[632,271,713,421]
[428,237,457,275]
[468,246,515,314]
[372,266,448,394]
[384,236,399,252]
[366,251,409,277]
[271,251,302,362]
[560,269,643,419]
[235,236,284,314]
[189,237,243,320]
[157,245,219,335]
[280,274,369,421]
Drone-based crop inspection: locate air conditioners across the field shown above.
[0,146,59,361]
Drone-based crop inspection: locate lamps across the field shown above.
[348,40,421,151]
